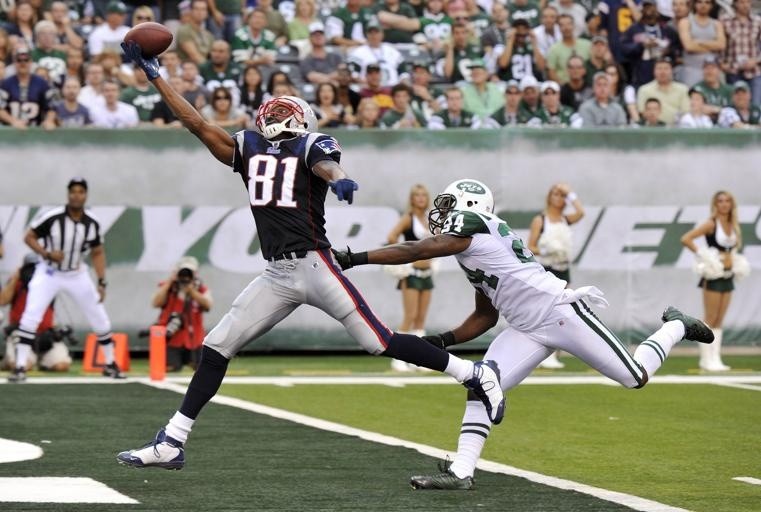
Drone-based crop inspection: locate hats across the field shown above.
[176,256,197,272]
[68,178,88,189]
[1,0,753,92]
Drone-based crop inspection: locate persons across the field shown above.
[151,255,213,372]
[526,183,585,369]
[329,177,717,492]
[387,183,433,372]
[6,176,127,383]
[0,254,73,373]
[681,191,743,373]
[115,38,506,471]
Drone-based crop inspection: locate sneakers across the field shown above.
[541,352,564,368]
[8,371,26,382]
[464,359,508,424]
[103,361,127,379]
[115,430,187,471]
[409,469,477,493]
[661,307,716,344]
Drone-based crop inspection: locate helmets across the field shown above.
[438,178,494,214]
[256,95,318,140]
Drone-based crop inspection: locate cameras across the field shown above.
[55,325,78,346]
[177,266,193,284]
[20,263,36,288]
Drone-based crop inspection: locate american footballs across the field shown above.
[124,21,172,58]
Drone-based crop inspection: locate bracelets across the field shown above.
[540,248,547,255]
[97,277,107,288]
[566,191,577,204]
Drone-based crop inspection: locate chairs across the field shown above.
[3,2,470,129]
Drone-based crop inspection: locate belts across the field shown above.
[265,250,307,263]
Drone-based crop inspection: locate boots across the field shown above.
[697,325,733,372]
[391,328,427,372]
